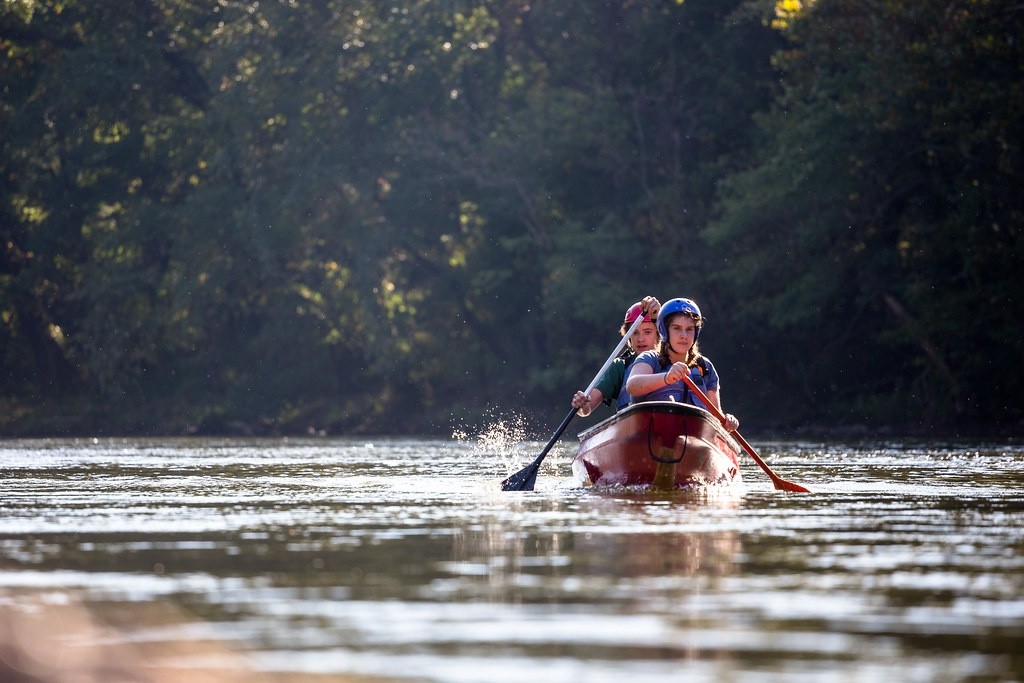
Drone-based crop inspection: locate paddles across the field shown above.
[681,372,810,493]
[499,305,658,491]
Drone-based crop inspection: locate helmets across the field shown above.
[657,298,702,342]
[625,302,654,323]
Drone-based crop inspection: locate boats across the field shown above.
[572,401,742,492]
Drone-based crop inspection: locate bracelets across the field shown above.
[664,372,671,386]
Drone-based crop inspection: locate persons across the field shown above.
[572,295,661,418]
[626,298,739,435]
